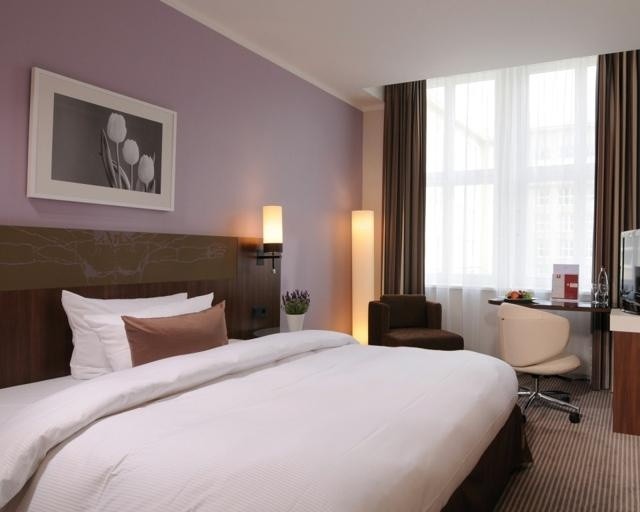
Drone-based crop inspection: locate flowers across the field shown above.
[279,288,312,314]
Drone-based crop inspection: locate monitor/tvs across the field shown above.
[620,229,640,315]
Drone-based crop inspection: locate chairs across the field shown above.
[496,302,583,424]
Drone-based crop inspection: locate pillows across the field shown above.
[120,299,229,368]
[82,291,215,372]
[60,289,191,381]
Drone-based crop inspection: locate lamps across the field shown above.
[256,205,284,273]
[350,210,375,346]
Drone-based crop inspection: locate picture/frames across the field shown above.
[26,67,177,212]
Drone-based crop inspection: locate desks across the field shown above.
[487,295,640,435]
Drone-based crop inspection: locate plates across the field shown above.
[495,295,536,301]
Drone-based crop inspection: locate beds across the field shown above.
[0,223,534,512]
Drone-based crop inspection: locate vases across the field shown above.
[287,314,306,332]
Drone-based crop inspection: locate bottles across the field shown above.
[597,267,608,300]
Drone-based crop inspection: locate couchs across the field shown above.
[367,294,464,351]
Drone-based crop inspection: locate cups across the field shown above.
[593,291,605,303]
